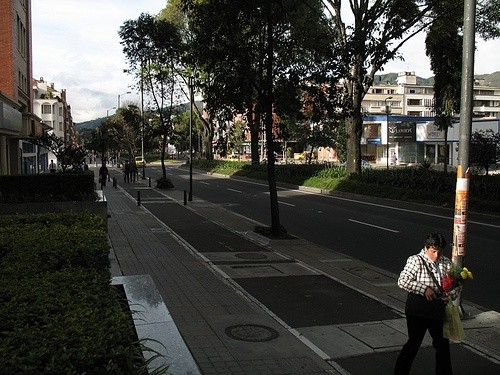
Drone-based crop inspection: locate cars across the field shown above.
[134,157,147,168]
[339,158,371,170]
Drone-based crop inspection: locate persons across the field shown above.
[393,232,463,375]
[123,160,131,183]
[99,162,108,190]
[129,160,137,182]
[49,160,56,173]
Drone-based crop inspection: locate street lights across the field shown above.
[127,105,147,180]
[384,95,394,169]
[106,106,117,118]
[117,91,131,109]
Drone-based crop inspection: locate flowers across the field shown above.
[446,264,473,282]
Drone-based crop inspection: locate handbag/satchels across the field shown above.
[421,297,446,316]
